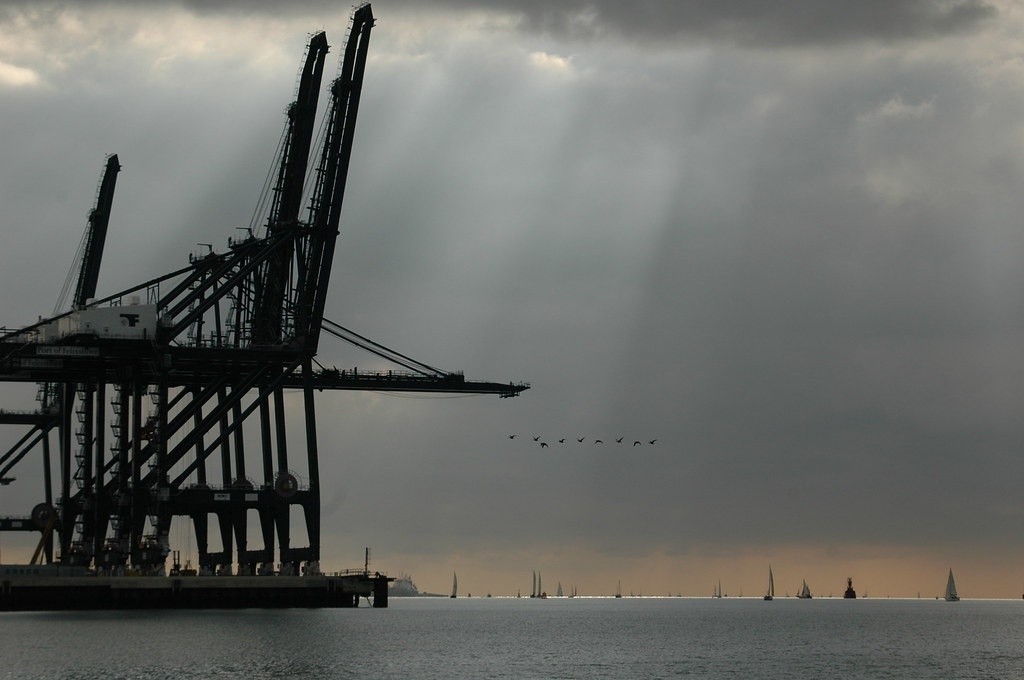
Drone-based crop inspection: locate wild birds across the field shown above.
[616,436,624,443]
[633,441,642,447]
[594,439,603,445]
[576,437,586,442]
[558,439,566,443]
[533,436,541,441]
[649,439,658,445]
[508,434,519,440]
[541,442,549,448]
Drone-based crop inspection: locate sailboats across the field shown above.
[799,579,812,599]
[615,579,621,598]
[530,572,536,598]
[536,571,542,598]
[764,564,775,601]
[557,582,563,597]
[943,567,961,601]
[450,570,457,598]
[568,585,577,598]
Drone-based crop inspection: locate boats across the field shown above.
[844,577,857,598]
[542,591,547,599]
